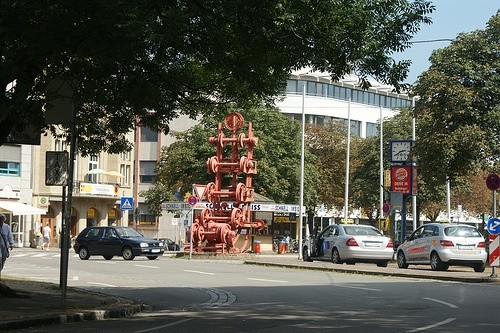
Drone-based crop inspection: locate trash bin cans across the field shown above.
[253,241,260,252]
[277,241,286,254]
[36,235,41,246]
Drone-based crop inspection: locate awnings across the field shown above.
[0,200,46,216]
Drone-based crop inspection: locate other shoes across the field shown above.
[47,249,49,250]
[41,245,44,249]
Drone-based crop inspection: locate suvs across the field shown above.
[74,226,165,261]
[396,223,488,272]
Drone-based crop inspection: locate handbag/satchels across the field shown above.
[7,250,9,257]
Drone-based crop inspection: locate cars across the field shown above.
[301,223,394,268]
[153,238,180,251]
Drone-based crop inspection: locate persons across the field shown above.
[0,213,14,271]
[41,222,51,251]
